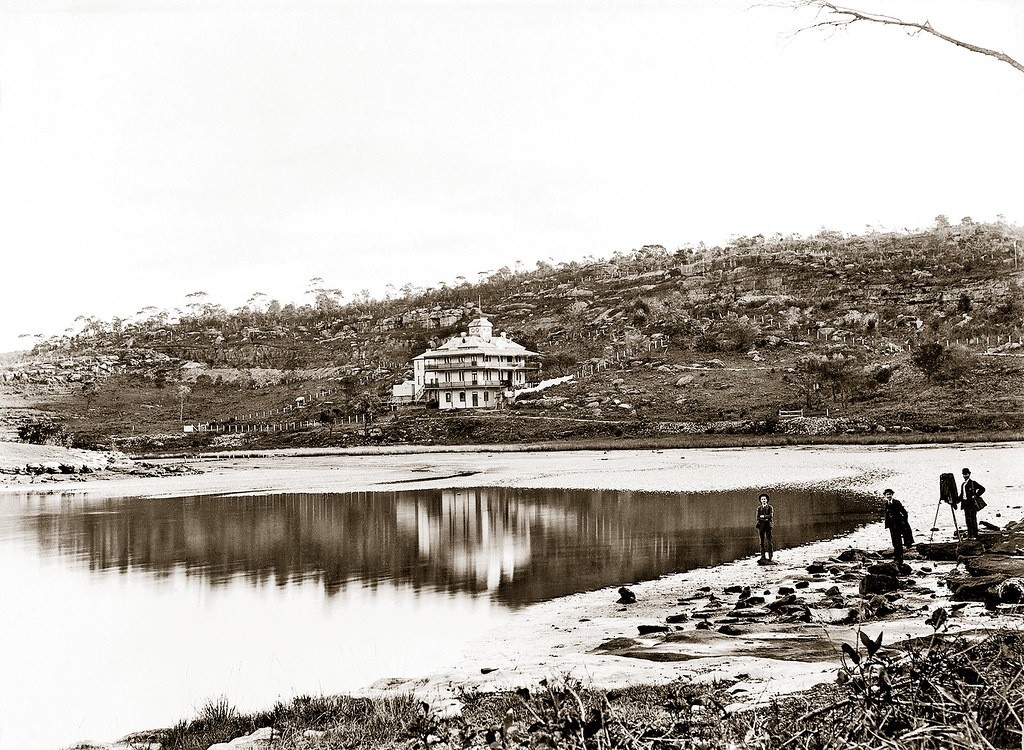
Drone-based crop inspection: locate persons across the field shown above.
[755,493,774,562]
[956,468,985,541]
[883,489,908,564]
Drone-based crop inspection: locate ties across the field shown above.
[889,501,891,505]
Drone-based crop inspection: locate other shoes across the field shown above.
[760,558,766,560]
[768,559,772,562]
[892,561,900,564]
[966,537,977,540]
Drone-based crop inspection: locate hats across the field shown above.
[759,494,769,502]
[961,468,971,474]
[883,489,895,495]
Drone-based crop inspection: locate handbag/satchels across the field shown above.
[973,497,987,511]
